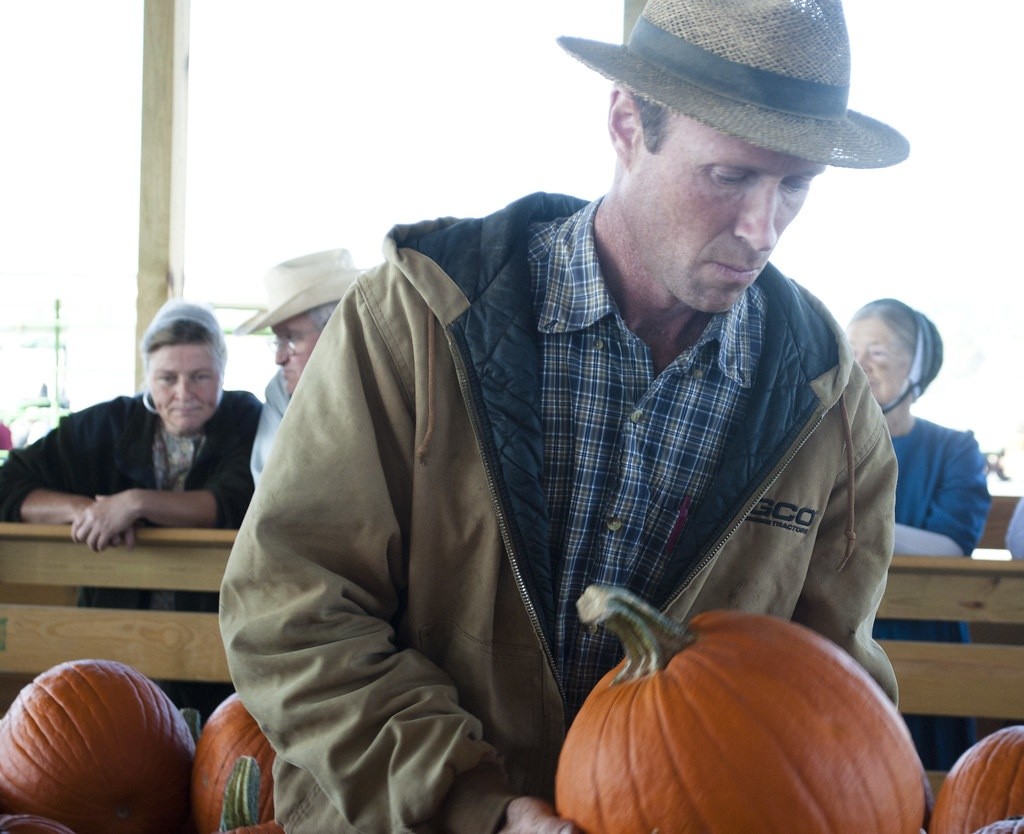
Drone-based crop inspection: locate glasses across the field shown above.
[263,321,327,353]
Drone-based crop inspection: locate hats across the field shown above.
[555,0,911,170]
[905,311,944,403]
[139,298,227,373]
[229,247,365,338]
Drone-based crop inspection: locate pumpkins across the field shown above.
[0,657,292,834]
[554,583,1024,834]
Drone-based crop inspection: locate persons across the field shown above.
[843,298,991,772]
[220,0,909,834]
[234,248,370,491]
[0,298,263,723]
